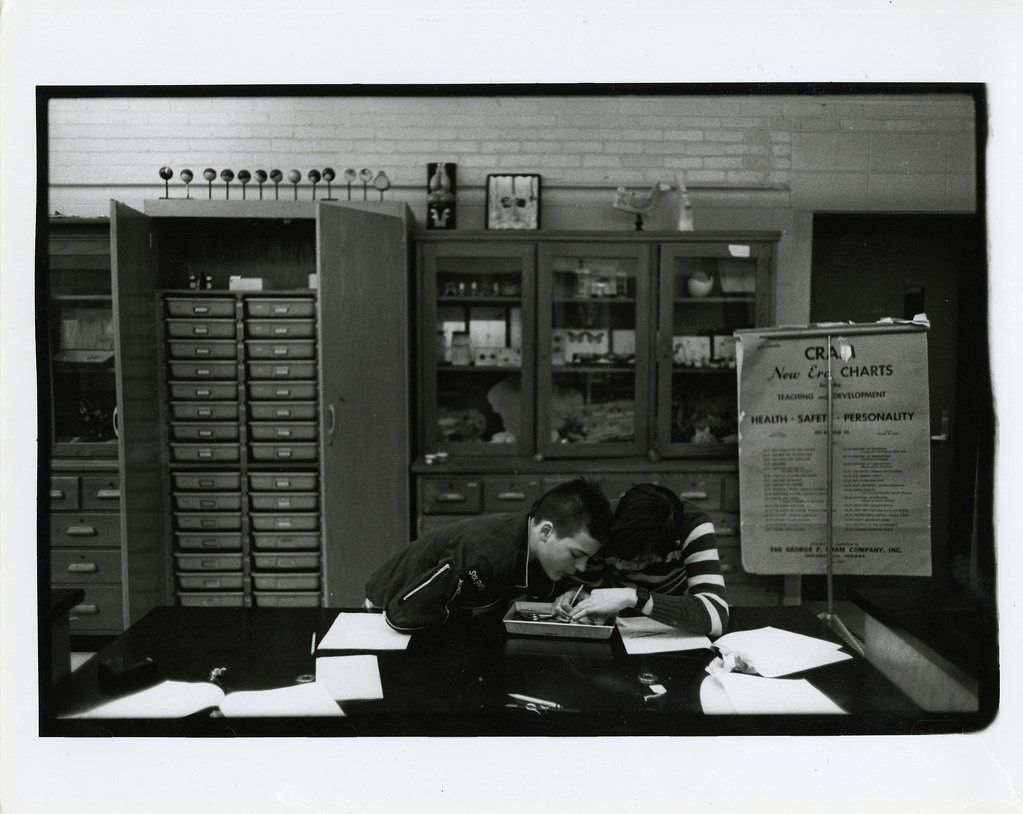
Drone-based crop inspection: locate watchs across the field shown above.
[634,586,650,613]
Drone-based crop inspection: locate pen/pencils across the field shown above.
[504,705,580,714]
[310,632,317,653]
[509,693,562,710]
[569,585,585,608]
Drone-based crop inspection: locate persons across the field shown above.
[551,483,730,637]
[364,475,613,635]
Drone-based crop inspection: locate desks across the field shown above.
[48,604,922,736]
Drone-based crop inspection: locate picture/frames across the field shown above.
[486,174,542,230]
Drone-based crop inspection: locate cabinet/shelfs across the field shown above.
[47,214,123,636]
[108,200,415,634]
[413,232,780,605]
